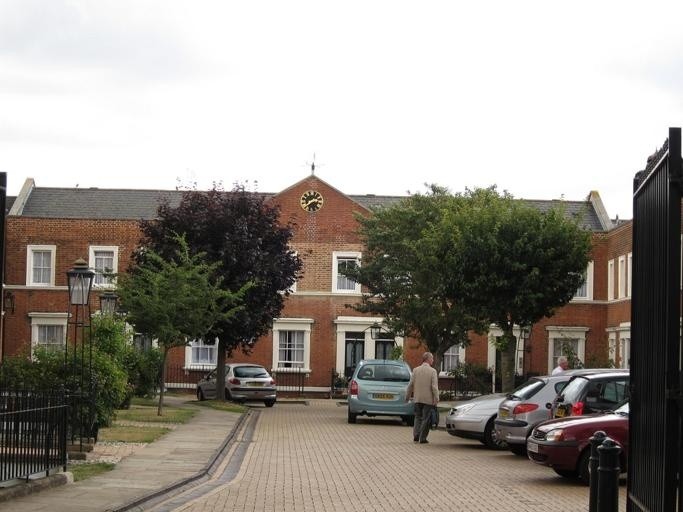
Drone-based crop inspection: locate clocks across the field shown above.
[299,190,323,213]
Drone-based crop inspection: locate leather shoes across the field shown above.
[420,439,428,443]
[414,436,419,442]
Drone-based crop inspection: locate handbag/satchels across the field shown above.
[429,405,440,430]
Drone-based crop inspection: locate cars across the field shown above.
[494,375,628,456]
[525,396,626,484]
[194,361,277,409]
[547,372,629,419]
[443,392,511,449]
[345,354,417,424]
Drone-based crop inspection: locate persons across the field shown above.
[406,352,440,443]
[551,355,568,375]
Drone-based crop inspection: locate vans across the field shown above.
[557,368,629,375]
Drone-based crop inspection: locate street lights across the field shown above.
[98,285,116,317]
[61,254,94,450]
